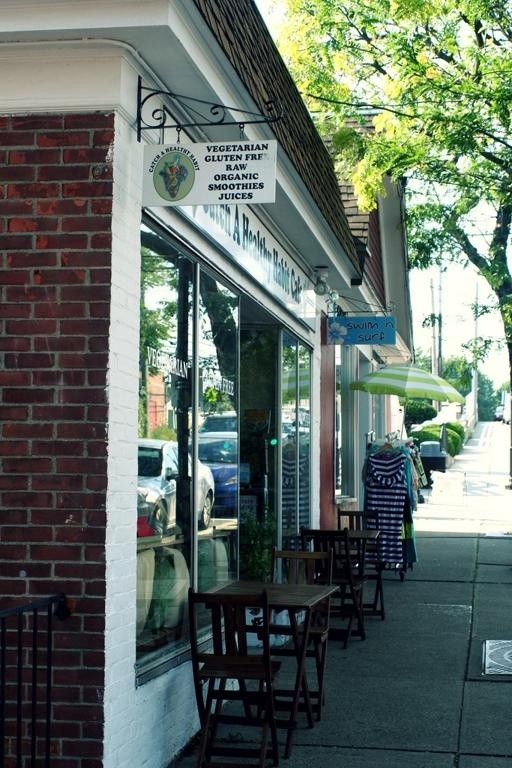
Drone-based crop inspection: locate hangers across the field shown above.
[377,430,402,453]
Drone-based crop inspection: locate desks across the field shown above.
[297,531,381,632]
[201,580,340,760]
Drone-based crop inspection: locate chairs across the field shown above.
[337,507,387,620]
[301,528,366,648]
[189,586,282,768]
[258,546,334,722]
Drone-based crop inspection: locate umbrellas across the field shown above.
[348,361,466,444]
[280,365,346,432]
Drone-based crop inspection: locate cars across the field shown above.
[138,437,216,537]
[188,433,238,518]
[200,411,238,434]
[281,404,301,433]
